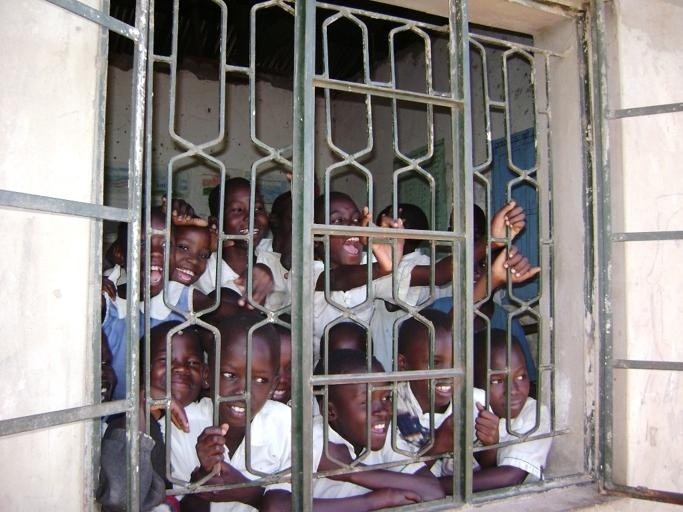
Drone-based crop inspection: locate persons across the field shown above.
[257,187,294,313]
[163,177,407,313]
[377,202,433,254]
[173,216,207,286]
[314,352,445,501]
[432,198,494,329]
[390,309,455,501]
[159,311,293,512]
[320,324,373,358]
[315,188,526,373]
[99,332,189,511]
[437,244,553,491]
[141,323,207,409]
[104,205,244,399]
[264,314,293,401]
